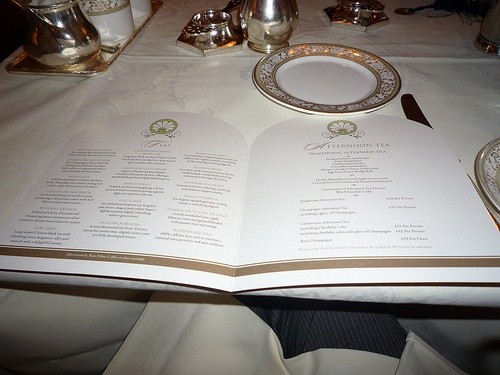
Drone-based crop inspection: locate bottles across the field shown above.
[475,0,500,56]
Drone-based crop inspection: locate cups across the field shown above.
[76,0,152,46]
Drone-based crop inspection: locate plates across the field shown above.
[252,43,402,116]
[474,138,500,225]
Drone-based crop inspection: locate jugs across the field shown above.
[238,0,295,54]
[23,2,100,68]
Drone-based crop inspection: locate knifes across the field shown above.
[400,94,432,130]
